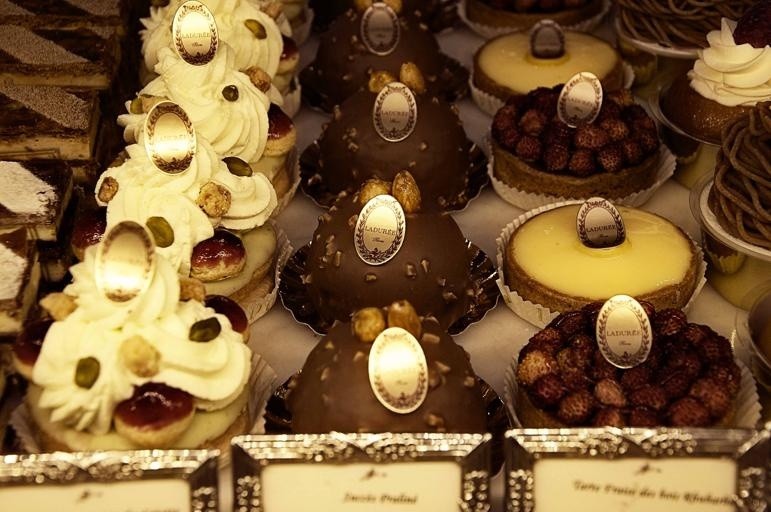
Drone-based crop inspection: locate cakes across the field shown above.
[0,1,771,452]
